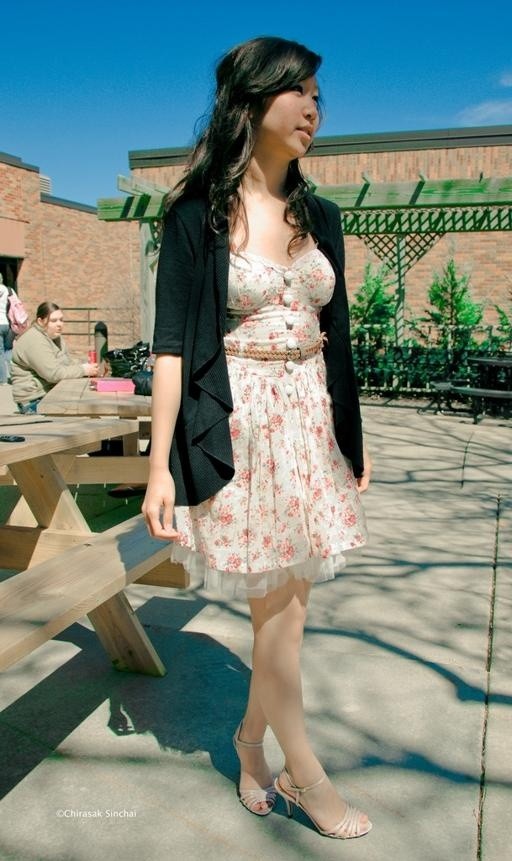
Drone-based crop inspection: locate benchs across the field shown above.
[450,386,512,424]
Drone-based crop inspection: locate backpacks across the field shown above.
[6,286,30,337]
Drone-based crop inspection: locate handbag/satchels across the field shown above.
[131,371,154,396]
[103,339,152,379]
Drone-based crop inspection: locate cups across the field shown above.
[88,349,97,378]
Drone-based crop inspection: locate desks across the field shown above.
[467,357,511,420]
[1,376,210,678]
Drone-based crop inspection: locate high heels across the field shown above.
[273,763,373,840]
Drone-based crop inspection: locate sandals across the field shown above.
[232,718,278,816]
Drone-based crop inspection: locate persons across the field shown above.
[8,303,145,455]
[143,35,375,839]
[0,270,18,384]
[105,433,151,498]
